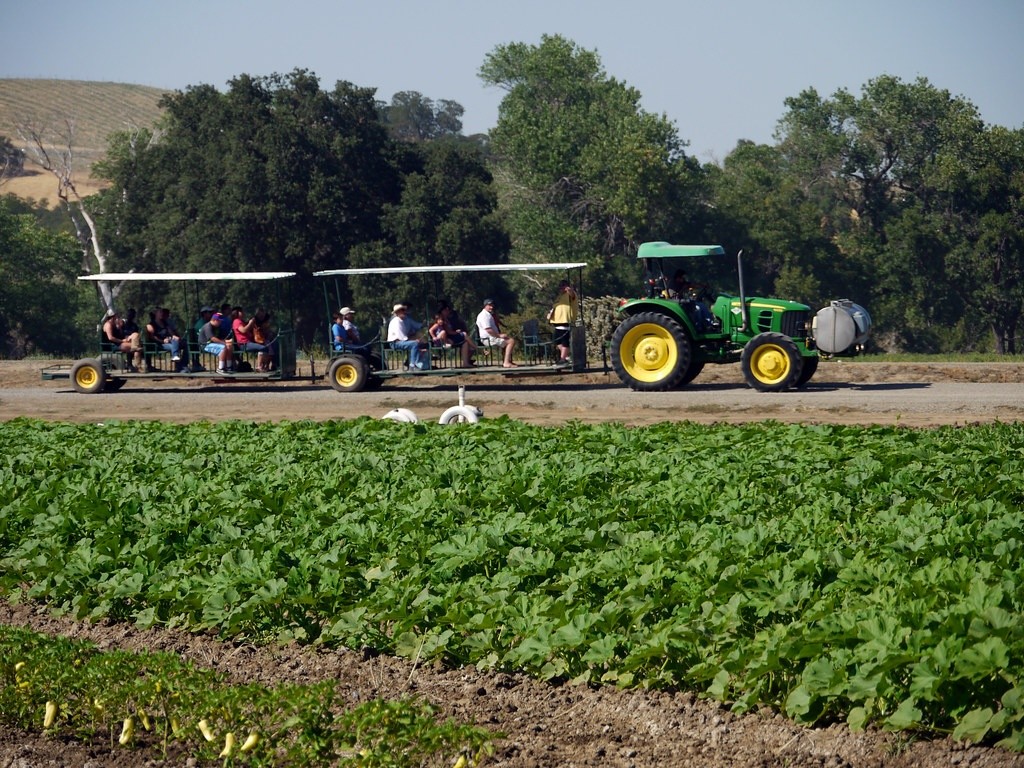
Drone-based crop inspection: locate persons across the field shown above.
[101,303,278,374]
[546,280,578,364]
[429,301,490,368]
[387,303,427,370]
[331,307,388,371]
[676,269,685,283]
[476,298,517,368]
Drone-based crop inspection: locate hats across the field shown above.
[340,307,355,315]
[200,306,214,313]
[484,299,493,305]
[212,312,226,321]
[391,304,407,314]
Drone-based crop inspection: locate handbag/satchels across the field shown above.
[254,323,265,344]
[547,308,556,319]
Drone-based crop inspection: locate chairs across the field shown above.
[330,321,558,372]
[96,325,277,374]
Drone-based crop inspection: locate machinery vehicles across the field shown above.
[608,241,873,393]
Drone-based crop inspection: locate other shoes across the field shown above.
[504,363,518,368]
[557,358,568,365]
[464,364,477,368]
[404,360,409,366]
[216,366,230,376]
[256,367,266,373]
[409,365,421,371]
[227,365,238,373]
[171,356,181,362]
[443,343,452,349]
[131,348,143,353]
[135,367,148,373]
